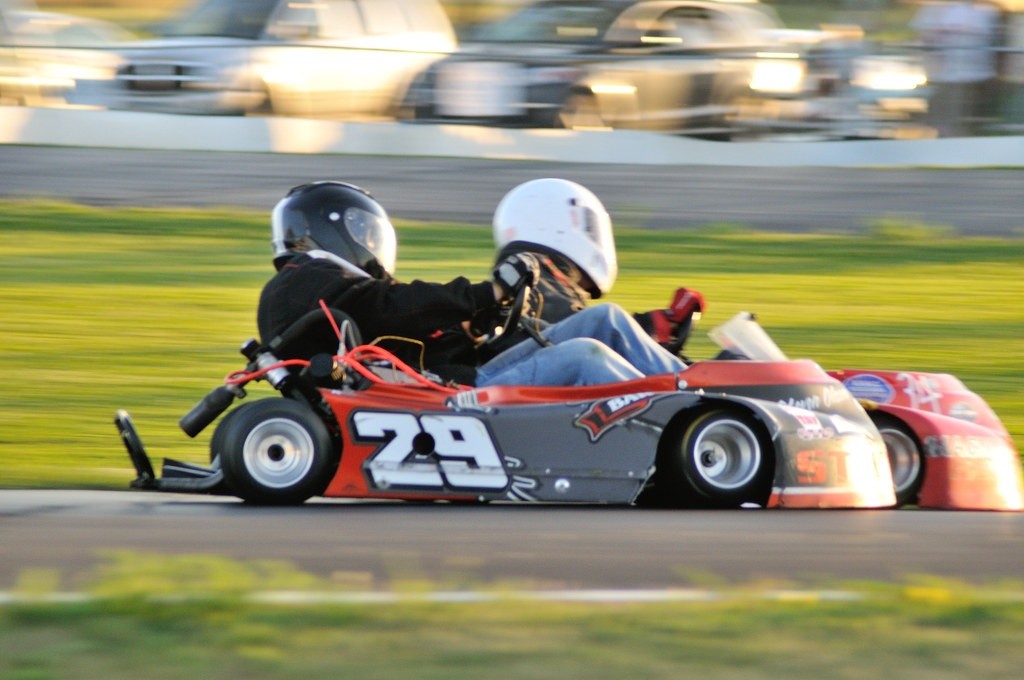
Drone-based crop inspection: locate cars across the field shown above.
[0,0,926,141]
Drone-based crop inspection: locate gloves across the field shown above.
[493,252,539,303]
[663,287,704,325]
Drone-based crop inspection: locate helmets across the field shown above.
[493,177,618,298]
[271,181,397,281]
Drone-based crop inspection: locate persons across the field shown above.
[912,0,996,137]
[256,179,691,385]
[490,176,705,349]
